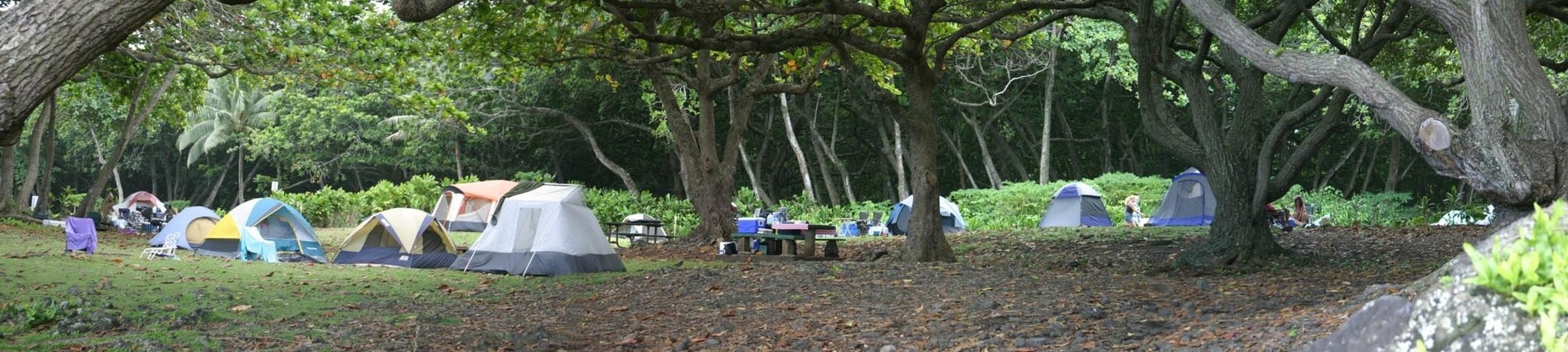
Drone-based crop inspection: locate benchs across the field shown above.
[731,233,846,257]
[608,233,679,248]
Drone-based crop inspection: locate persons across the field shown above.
[98,191,176,233]
[1119,195,1145,228]
[1266,197,1309,228]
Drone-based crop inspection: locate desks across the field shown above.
[771,207,835,257]
[606,223,664,248]
[836,217,854,238]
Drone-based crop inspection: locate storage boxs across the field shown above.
[738,217,766,233]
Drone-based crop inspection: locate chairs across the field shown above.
[139,233,182,261]
[858,212,883,236]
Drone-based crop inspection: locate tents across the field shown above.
[611,213,669,243]
[1036,181,1113,228]
[885,194,966,237]
[194,198,329,264]
[146,206,222,250]
[330,207,458,269]
[448,180,628,278]
[431,179,518,232]
[1144,166,1216,226]
